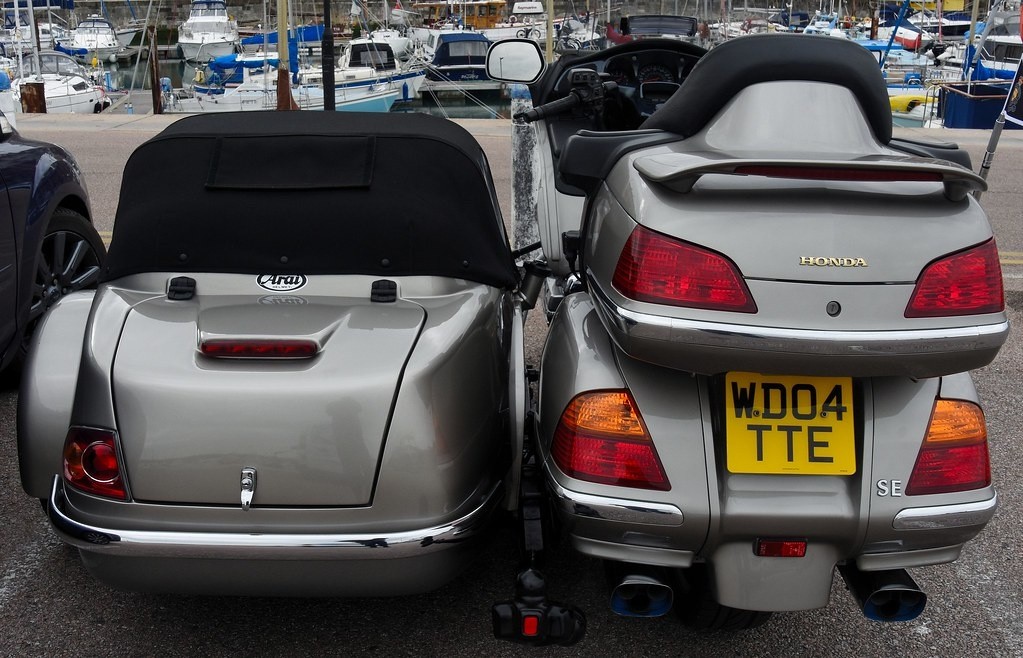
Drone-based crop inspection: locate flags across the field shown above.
[101,0,108,18]
[351,2,362,16]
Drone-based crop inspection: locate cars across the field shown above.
[0,102,111,377]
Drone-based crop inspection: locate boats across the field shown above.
[0,0,1023,129]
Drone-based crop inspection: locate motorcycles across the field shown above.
[12,20,1011,645]
